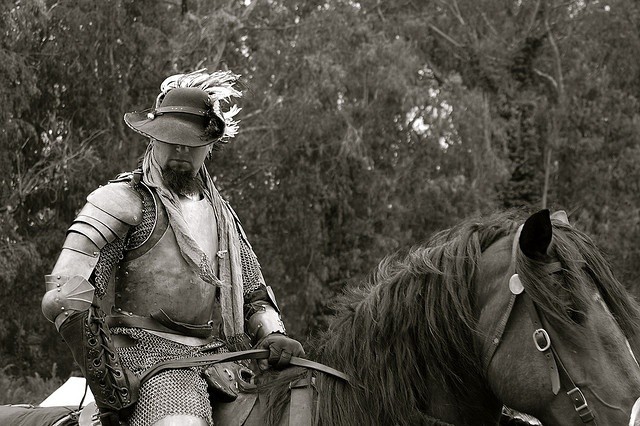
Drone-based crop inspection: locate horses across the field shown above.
[0,208,640,426]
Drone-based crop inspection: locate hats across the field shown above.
[124,88,226,148]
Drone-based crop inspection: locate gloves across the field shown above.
[257,332,306,372]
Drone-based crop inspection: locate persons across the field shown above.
[41,67,305,425]
